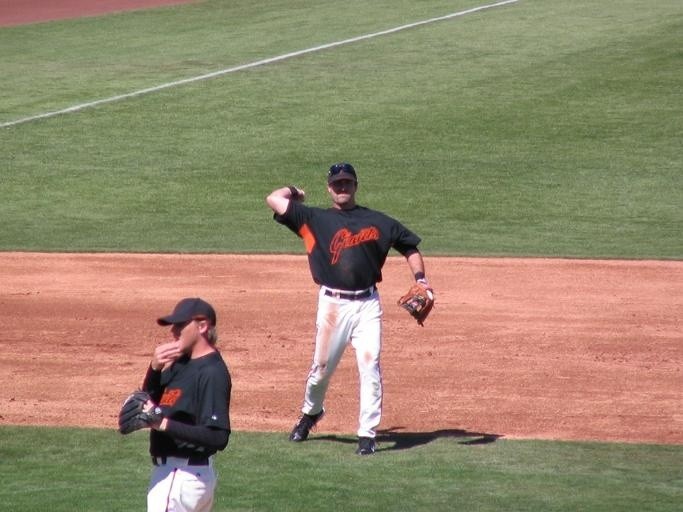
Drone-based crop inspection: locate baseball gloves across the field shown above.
[398,283,435,328]
[118,391,164,435]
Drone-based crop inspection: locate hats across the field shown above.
[157,298,216,326]
[326,162,358,185]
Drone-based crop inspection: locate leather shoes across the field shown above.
[356,436,376,457]
[289,409,325,441]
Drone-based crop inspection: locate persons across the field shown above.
[118,297,232,512]
[266,163,434,454]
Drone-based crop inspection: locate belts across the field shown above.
[152,456,210,466]
[320,283,377,302]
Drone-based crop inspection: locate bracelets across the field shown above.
[415,271,425,282]
[287,185,298,199]
